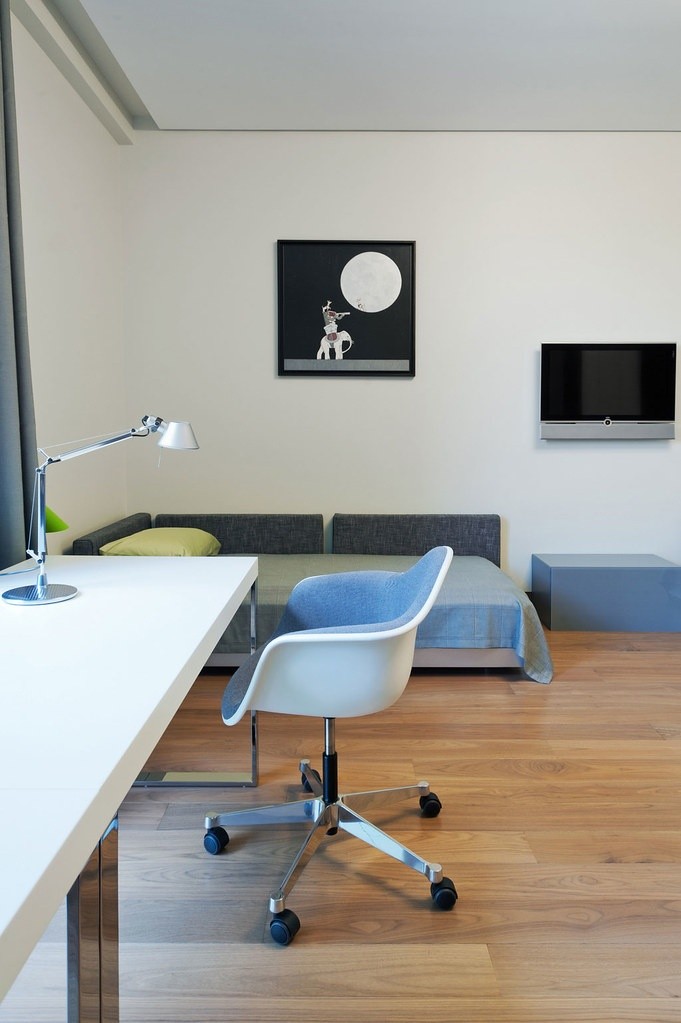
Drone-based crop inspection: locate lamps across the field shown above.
[3,414,199,606]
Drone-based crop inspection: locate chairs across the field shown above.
[203,544,460,946]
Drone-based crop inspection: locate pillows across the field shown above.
[99,526,222,557]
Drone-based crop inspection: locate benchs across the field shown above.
[529,553,681,634]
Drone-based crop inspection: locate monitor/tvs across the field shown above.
[539,343,677,440]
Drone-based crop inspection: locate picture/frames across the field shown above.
[276,239,415,376]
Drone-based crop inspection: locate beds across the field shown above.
[69,512,557,686]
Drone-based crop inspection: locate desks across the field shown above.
[0,544,258,1023]
